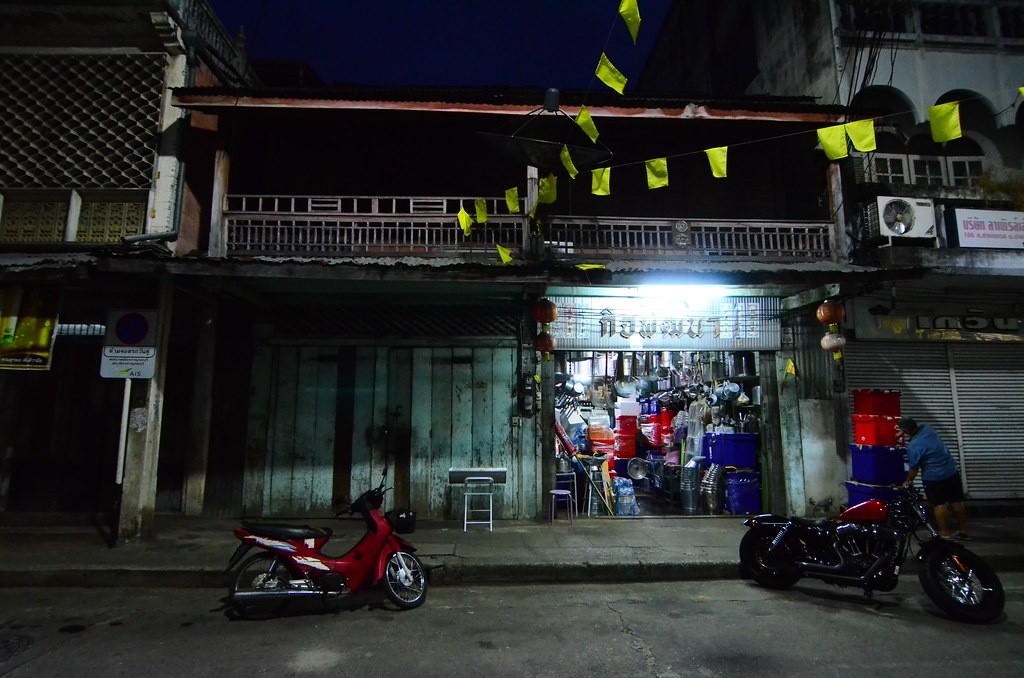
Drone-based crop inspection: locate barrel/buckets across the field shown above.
[680,463,726,516]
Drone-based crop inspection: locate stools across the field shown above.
[556,472,578,518]
[549,489,573,527]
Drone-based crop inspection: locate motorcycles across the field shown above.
[739,484,1006,623]
[226,465,429,621]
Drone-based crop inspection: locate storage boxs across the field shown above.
[848,444,907,486]
[615,415,637,434]
[614,434,635,458]
[851,414,902,446]
[851,387,900,416]
[702,433,759,469]
[845,481,906,507]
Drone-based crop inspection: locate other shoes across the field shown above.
[926,530,949,539]
[952,532,972,541]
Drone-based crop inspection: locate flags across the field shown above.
[644,157,669,190]
[505,187,520,214]
[928,101,962,142]
[618,0,641,43]
[538,177,557,203]
[591,167,611,196]
[576,264,605,271]
[595,52,628,95]
[456,207,473,236]
[495,244,512,263]
[574,104,600,144]
[704,145,727,178]
[816,118,877,161]
[560,144,579,180]
[474,197,488,224]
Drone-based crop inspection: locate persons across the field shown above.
[898,415,969,540]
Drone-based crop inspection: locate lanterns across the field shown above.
[817,301,845,332]
[820,331,845,360]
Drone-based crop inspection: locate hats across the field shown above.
[899,416,916,428]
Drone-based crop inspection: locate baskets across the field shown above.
[383,506,417,535]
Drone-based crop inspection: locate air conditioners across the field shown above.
[867,196,937,241]
